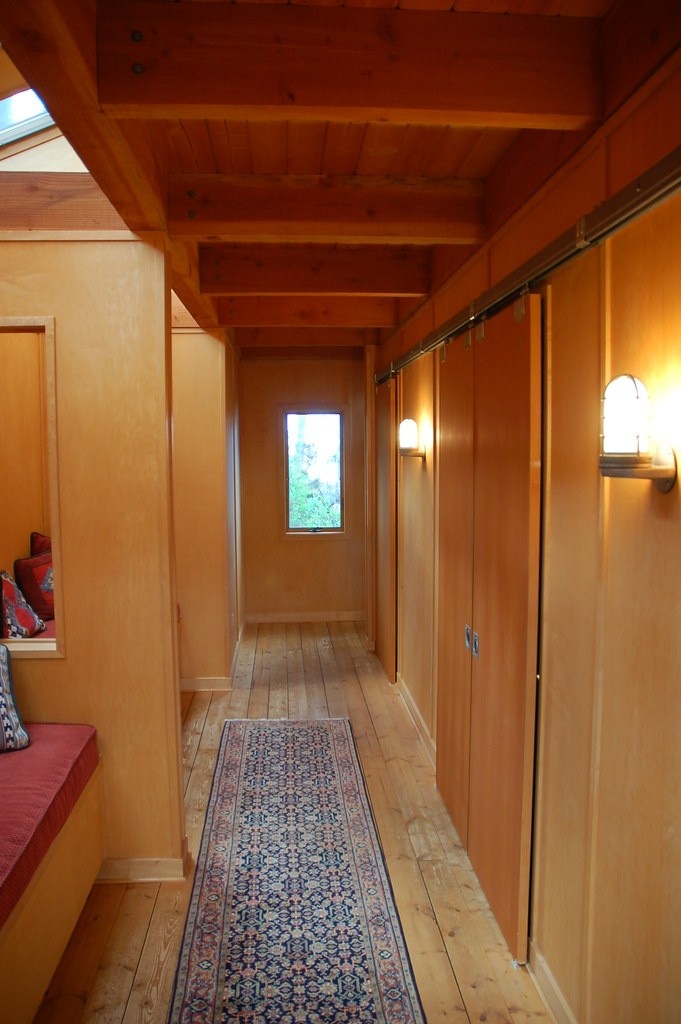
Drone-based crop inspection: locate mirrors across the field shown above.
[0,316,67,658]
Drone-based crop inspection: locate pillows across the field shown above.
[0,531,55,639]
[0,644,30,753]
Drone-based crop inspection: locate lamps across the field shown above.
[597,374,676,492]
[398,418,427,457]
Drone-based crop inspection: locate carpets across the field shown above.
[166,718,427,1024]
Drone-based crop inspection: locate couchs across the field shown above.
[0,724,104,1024]
